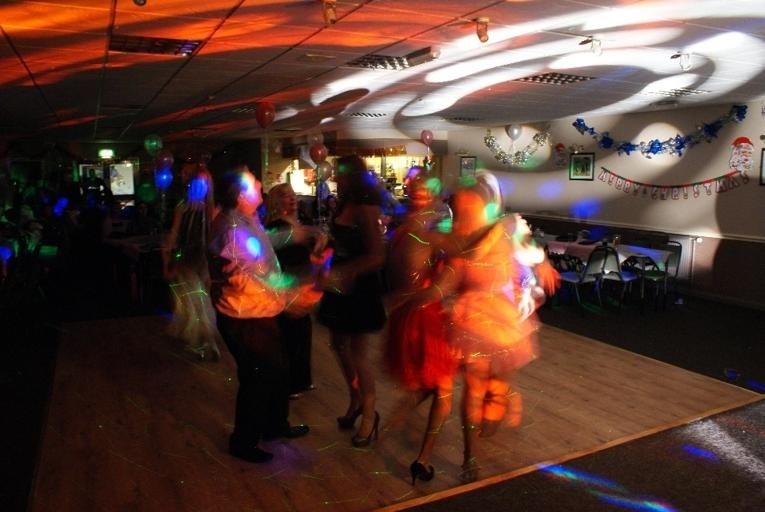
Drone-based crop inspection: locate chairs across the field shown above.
[543,240,682,323]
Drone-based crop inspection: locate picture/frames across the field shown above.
[759,148,765,185]
[459,156,476,177]
[569,152,595,181]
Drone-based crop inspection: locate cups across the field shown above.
[613,235,622,249]
[603,239,608,247]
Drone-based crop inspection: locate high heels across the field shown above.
[351,410,380,448]
[336,404,363,427]
[410,459,434,486]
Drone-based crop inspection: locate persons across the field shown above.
[162,154,560,486]
[85,169,101,187]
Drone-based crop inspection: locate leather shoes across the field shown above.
[478,420,499,438]
[262,424,310,441]
[229,444,274,463]
